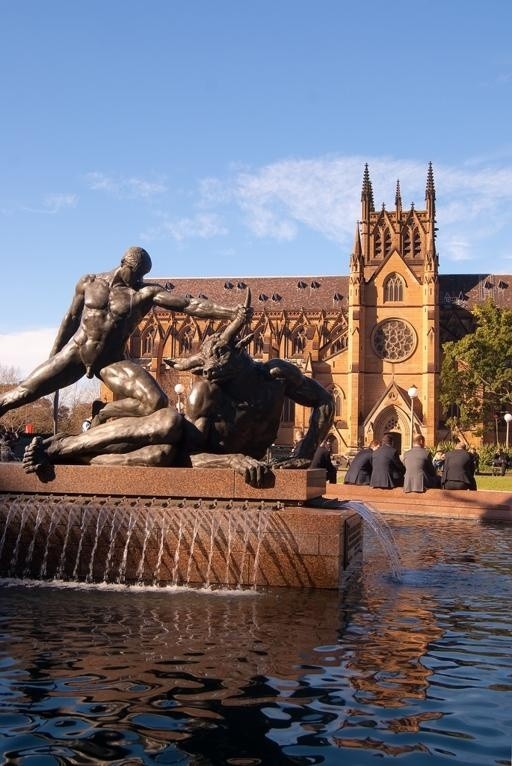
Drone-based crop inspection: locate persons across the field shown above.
[80,415,92,432]
[291,429,510,492]
[0,246,334,486]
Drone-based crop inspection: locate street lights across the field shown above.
[503,412,512,450]
[407,385,418,449]
[174,383,185,413]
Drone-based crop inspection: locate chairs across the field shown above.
[492,457,512,476]
